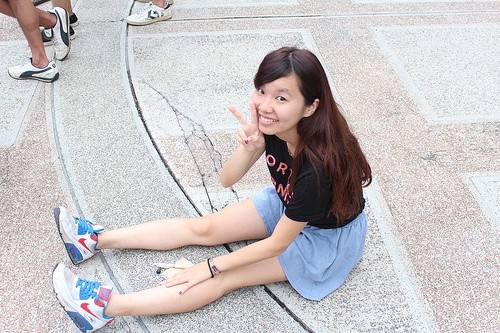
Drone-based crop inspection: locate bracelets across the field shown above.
[207,258,220,278]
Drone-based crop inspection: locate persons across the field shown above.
[127,0,174,26]
[0,0,79,82]
[52,47,372,333]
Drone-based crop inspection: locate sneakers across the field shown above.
[53,262,115,333]
[54,206,105,265]
[137,0,174,4]
[43,6,71,61]
[8,58,59,84]
[127,2,173,26]
[69,13,79,27]
[40,26,76,47]
